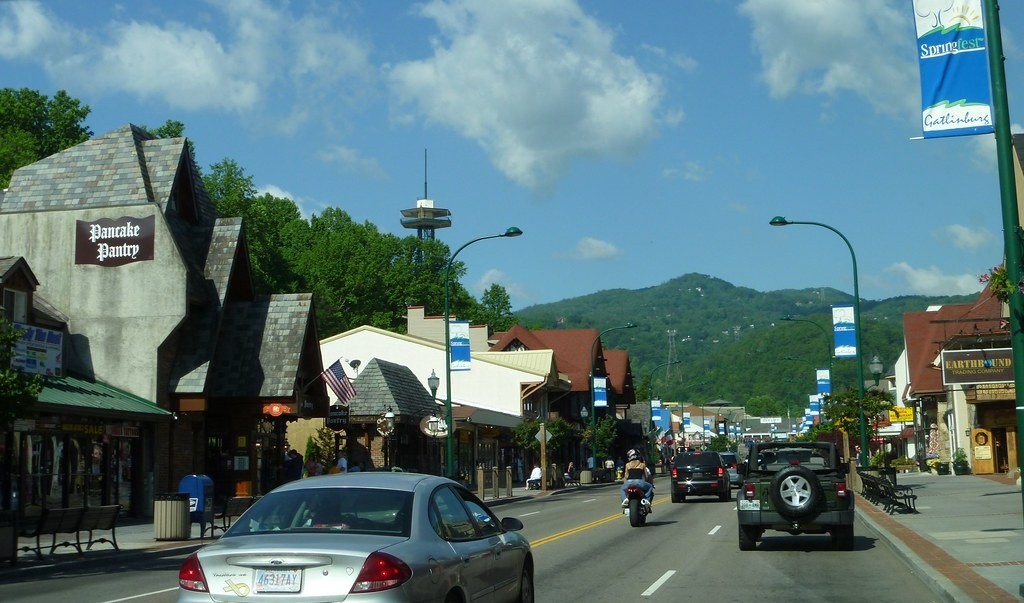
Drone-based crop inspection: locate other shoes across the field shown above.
[621,499,629,507]
[647,507,652,513]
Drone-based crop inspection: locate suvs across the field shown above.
[738,440,857,550]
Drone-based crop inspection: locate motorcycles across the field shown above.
[623,485,651,527]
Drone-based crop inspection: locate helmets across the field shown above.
[626,449,640,461]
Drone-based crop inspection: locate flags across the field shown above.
[650,427,686,448]
[320,360,356,404]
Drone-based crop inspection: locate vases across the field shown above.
[934,461,949,475]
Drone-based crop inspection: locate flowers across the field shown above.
[975,266,1024,303]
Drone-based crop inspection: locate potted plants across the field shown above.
[952,448,967,475]
[890,458,917,470]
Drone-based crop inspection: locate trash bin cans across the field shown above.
[178,474,215,538]
[154,492,191,541]
[879,467,897,487]
[856,466,879,494]
[579,468,592,484]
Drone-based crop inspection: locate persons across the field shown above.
[855,445,863,466]
[620,449,653,508]
[525,455,653,495]
[286,449,363,484]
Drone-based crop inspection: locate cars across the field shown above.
[175,470,534,603]
[671,452,731,502]
[718,451,744,486]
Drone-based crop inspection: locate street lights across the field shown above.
[444,227,525,474]
[589,322,640,486]
[768,216,868,467]
[647,360,684,479]
[780,315,834,431]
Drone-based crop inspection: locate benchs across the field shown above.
[205,495,264,532]
[564,468,583,487]
[16,504,125,561]
[528,467,554,491]
[858,473,919,516]
